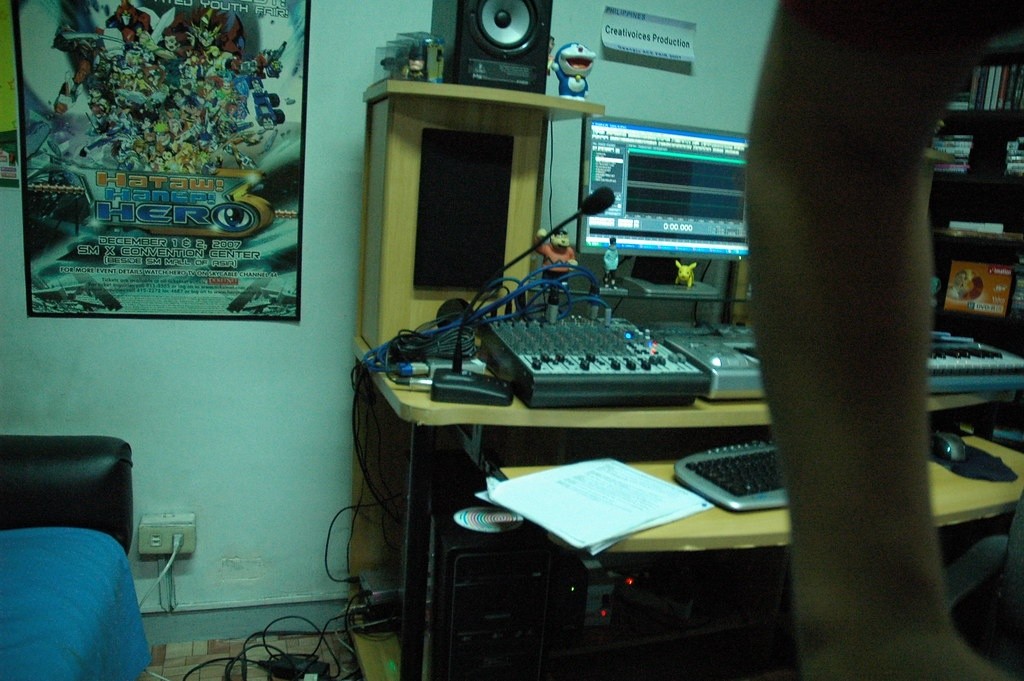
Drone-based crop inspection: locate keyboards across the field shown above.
[672,441,790,511]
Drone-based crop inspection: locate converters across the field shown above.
[271,656,330,681]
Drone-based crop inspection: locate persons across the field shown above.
[533,229,579,291]
[742,0,1024,681]
[602,238,620,289]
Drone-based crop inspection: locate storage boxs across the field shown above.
[942,259,1015,317]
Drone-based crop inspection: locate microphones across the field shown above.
[429,187,616,406]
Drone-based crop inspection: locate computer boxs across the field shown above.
[405,447,551,681]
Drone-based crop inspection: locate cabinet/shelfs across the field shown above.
[345,22,1024,681]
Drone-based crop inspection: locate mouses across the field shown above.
[930,431,967,465]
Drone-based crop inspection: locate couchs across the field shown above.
[0,434,135,681]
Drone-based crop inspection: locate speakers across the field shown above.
[428,0,553,94]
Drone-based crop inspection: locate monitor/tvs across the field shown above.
[576,115,748,297]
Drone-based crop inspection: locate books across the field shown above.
[929,61,1024,317]
